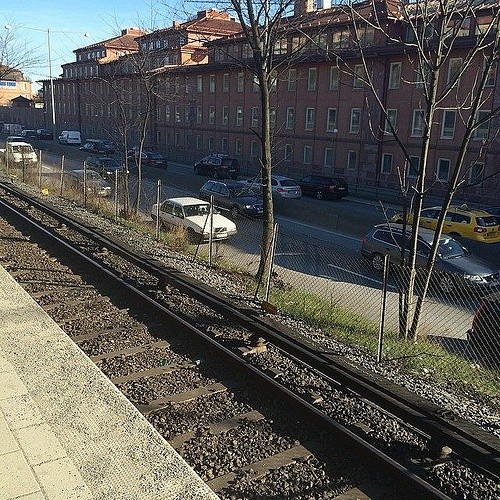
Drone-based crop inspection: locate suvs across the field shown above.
[198,180,266,217]
[295,175,350,199]
[57,130,82,145]
[363,225,500,295]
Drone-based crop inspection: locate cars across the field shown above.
[0,121,37,148]
[395,205,500,245]
[62,170,114,198]
[151,197,239,245]
[242,176,301,199]
[83,157,130,182]
[79,139,116,154]
[465,292,500,357]
[124,145,168,169]
[36,129,54,140]
[194,153,240,178]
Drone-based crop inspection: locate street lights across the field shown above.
[13,26,90,126]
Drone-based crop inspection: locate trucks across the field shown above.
[0,142,38,169]
